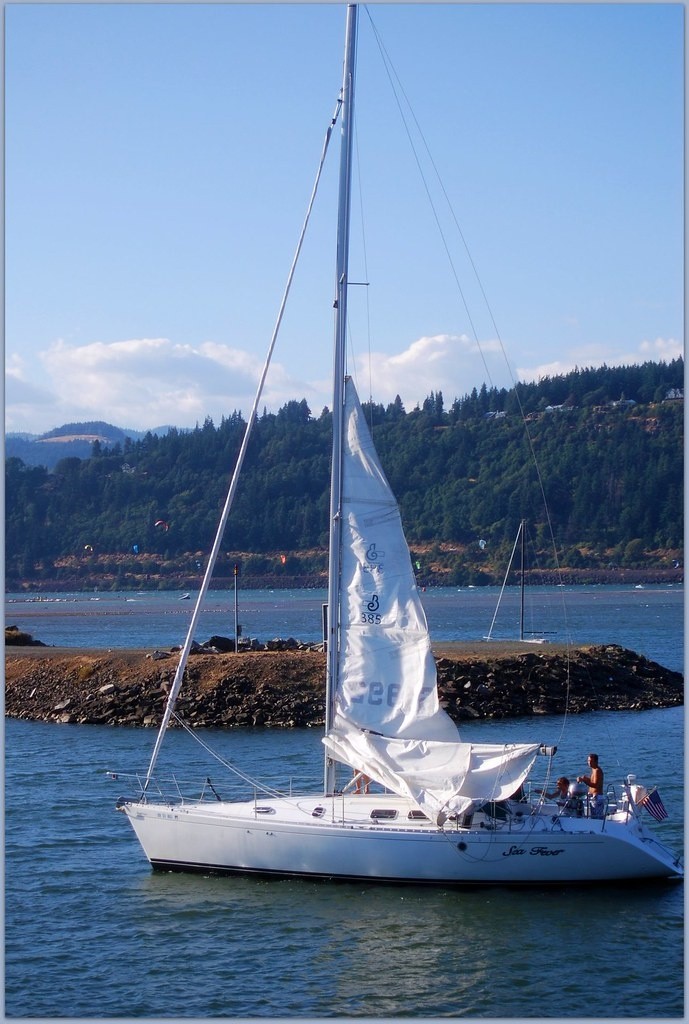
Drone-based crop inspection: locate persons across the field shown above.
[351,768,370,794]
[577,753,605,819]
[534,777,584,818]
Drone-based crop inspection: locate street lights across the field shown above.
[232,564,239,653]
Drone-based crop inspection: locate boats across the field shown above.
[178,592,191,600]
[636,585,644,589]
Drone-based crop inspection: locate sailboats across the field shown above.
[105,6,683,888]
[484,519,557,644]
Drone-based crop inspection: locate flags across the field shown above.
[641,790,668,823]
